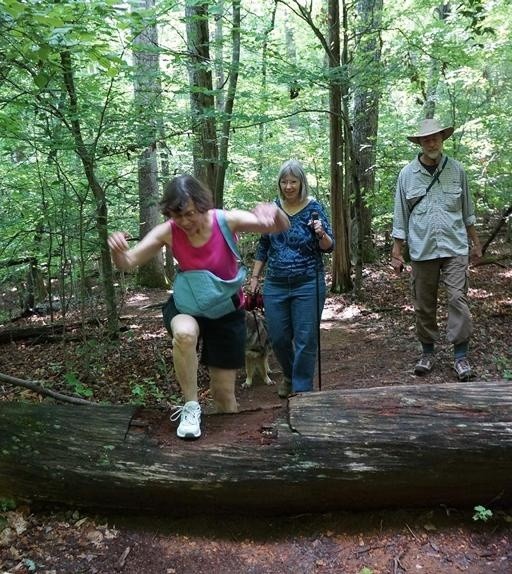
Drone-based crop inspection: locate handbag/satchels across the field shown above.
[173,266,248,320]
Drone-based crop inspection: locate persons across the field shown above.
[248,159,336,400]
[389,118,487,386]
[106,176,290,443]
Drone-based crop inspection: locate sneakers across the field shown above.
[277,376,293,397]
[169,400,202,438]
[453,357,472,380]
[414,352,436,374]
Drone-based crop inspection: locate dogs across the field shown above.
[241,307,277,389]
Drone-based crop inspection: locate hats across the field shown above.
[407,119,455,146]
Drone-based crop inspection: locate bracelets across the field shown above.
[250,275,259,279]
